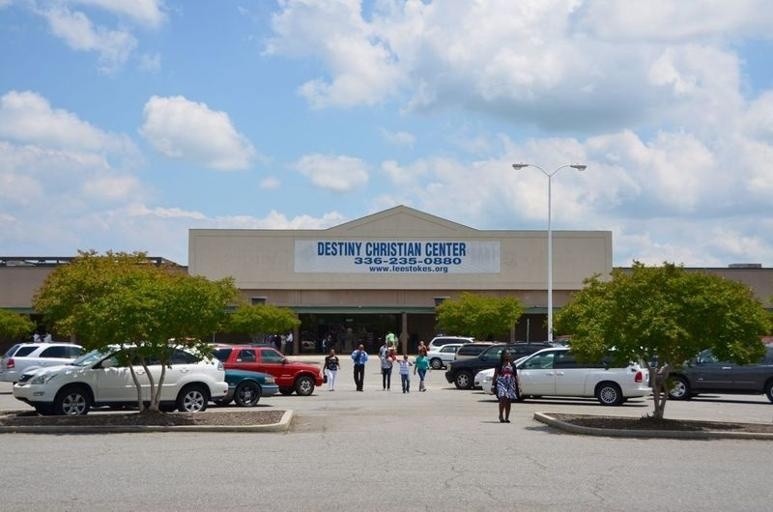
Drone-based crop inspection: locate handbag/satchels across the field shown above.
[323,372,327,383]
[352,350,359,359]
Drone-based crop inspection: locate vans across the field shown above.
[480,343,652,406]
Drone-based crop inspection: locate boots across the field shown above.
[419,380,427,391]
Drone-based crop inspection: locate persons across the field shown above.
[322,349,340,391]
[268,316,409,355]
[351,344,368,391]
[491,348,519,423]
[387,341,396,361]
[396,354,412,394]
[413,349,430,391]
[377,342,386,359]
[380,351,393,390]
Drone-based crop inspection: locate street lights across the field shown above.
[510,160,589,345]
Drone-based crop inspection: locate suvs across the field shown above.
[423,331,668,386]
[656,338,772,405]
